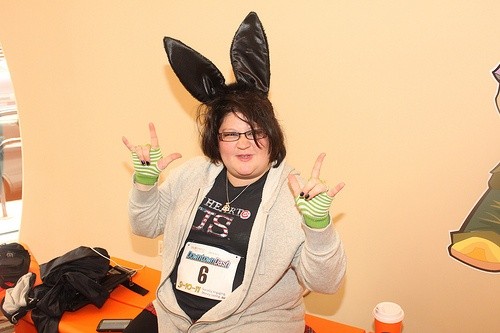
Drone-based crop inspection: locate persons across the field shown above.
[122,12,346,333]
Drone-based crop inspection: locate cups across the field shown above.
[373,302,406,333]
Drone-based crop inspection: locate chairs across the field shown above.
[0,100,23,218]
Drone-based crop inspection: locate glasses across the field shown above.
[218,129,268,142]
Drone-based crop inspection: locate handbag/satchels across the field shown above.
[0,243,31,288]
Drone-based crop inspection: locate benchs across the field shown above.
[0,255,366,333]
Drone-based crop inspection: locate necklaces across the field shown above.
[222,172,260,211]
